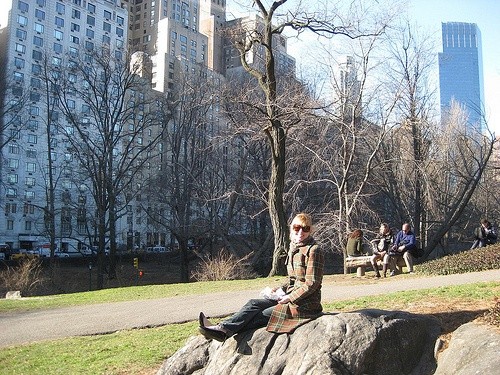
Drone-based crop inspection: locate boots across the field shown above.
[372,263,380,277]
[383,264,388,277]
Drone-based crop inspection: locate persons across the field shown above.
[346,228,364,257]
[370,222,420,278]
[197,213,324,342]
[469,218,498,249]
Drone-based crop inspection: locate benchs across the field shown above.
[345,256,406,277]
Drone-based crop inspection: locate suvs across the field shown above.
[0,244,10,260]
[81,246,108,255]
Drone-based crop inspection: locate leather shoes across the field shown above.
[199,312,213,326]
[198,325,227,342]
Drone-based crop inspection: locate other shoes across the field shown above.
[408,269,412,272]
[390,270,395,276]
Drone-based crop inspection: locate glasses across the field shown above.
[293,224,310,232]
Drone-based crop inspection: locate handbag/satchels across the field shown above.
[389,243,404,256]
[475,227,485,240]
[377,239,387,252]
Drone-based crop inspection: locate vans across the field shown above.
[146,247,170,253]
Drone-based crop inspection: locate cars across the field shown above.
[46,250,69,257]
[11,251,40,261]
